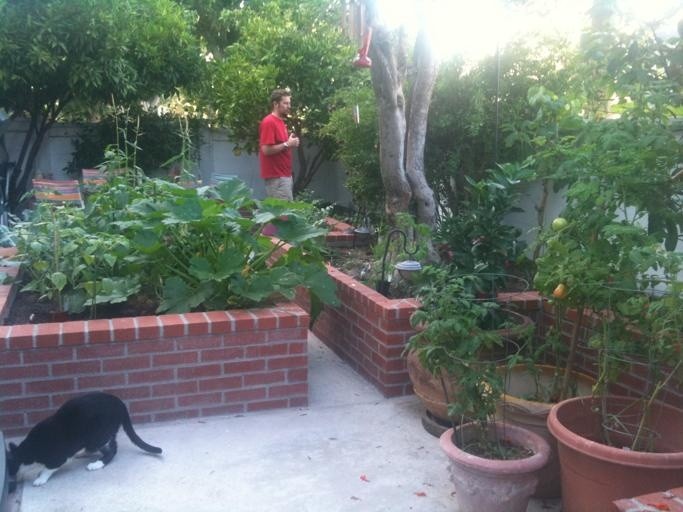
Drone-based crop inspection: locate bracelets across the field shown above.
[283,143,288,150]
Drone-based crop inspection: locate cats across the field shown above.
[5,391,162,486]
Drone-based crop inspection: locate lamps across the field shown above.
[375,228,421,295]
[352,200,373,255]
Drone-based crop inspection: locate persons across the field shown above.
[259,90,299,202]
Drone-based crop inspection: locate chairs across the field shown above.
[30,175,85,211]
[80,165,125,195]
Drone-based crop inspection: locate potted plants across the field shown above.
[0,91,343,441]
